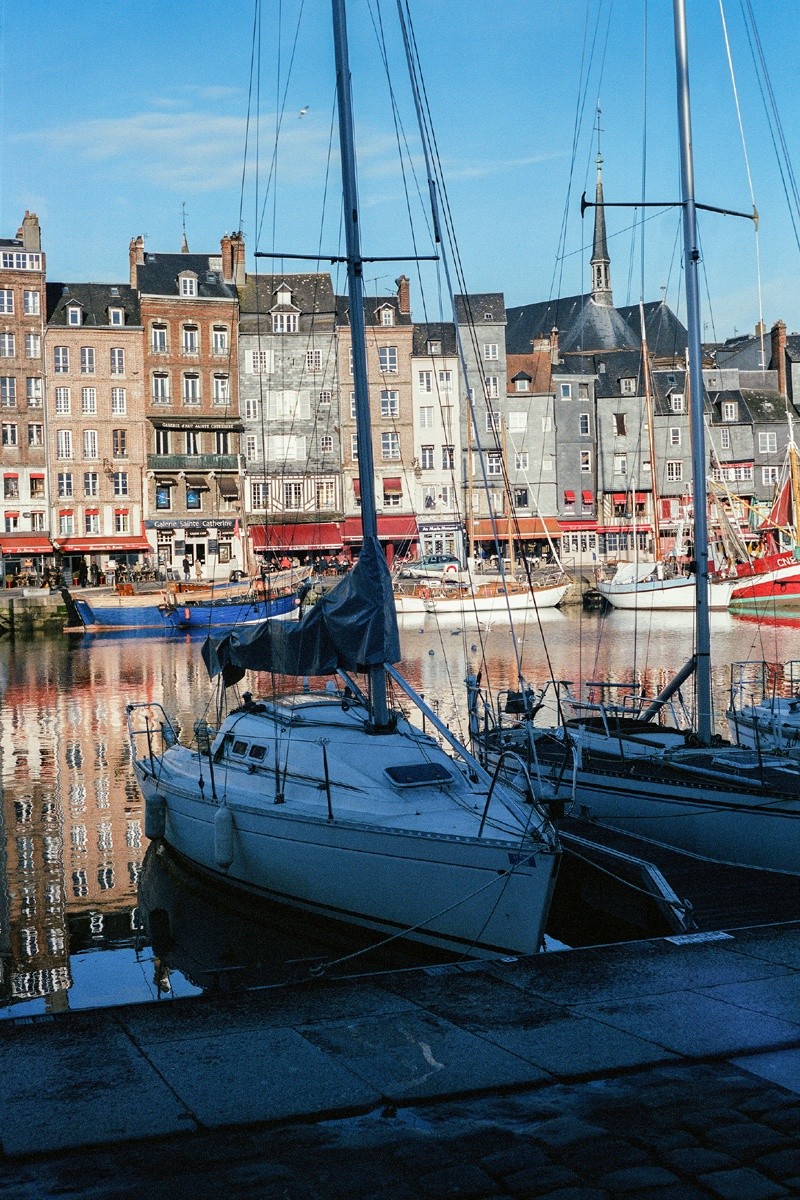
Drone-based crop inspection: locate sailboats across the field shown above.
[123,0,799,961]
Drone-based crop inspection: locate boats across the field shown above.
[59,564,316,634]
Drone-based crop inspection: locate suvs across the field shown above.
[396,553,461,579]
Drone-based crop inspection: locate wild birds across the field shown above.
[421,621,528,655]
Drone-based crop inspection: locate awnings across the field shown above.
[52,536,150,554]
[251,523,344,549]
[0,531,53,553]
[341,516,420,541]
[465,518,562,540]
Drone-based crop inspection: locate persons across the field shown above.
[7,542,559,591]
